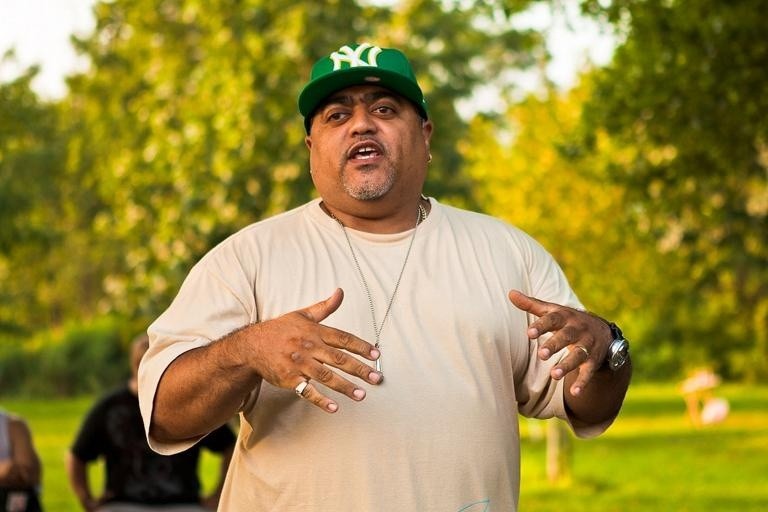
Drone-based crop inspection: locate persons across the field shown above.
[64,326,234,512]
[134,41,635,511]
[0,408,47,512]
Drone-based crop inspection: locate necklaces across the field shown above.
[330,201,428,375]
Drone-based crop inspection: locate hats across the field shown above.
[299,44,428,134]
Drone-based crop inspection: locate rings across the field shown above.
[293,381,309,395]
[576,344,589,361]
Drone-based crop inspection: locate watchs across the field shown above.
[602,319,630,373]
